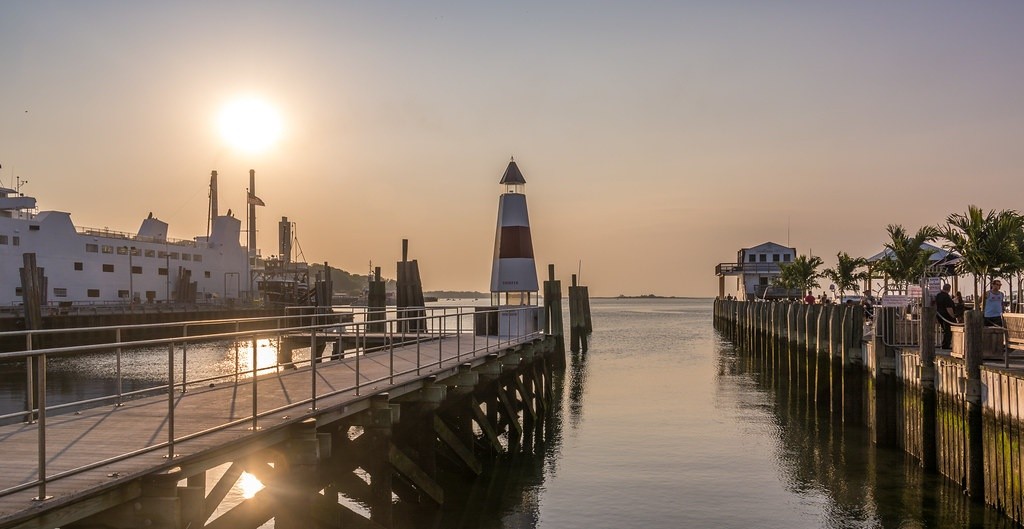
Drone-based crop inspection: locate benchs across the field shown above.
[1001,312,1024,367]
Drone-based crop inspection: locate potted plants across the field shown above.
[935,205,1024,359]
[872,223,939,344]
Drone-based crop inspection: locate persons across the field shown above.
[936,284,957,350]
[863,290,876,321]
[727,293,732,300]
[978,280,1004,327]
[822,291,827,299]
[952,291,965,323]
[835,296,841,304]
[805,291,815,305]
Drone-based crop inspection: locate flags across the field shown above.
[246,192,265,206]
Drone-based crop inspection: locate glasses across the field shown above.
[993,283,1001,286]
